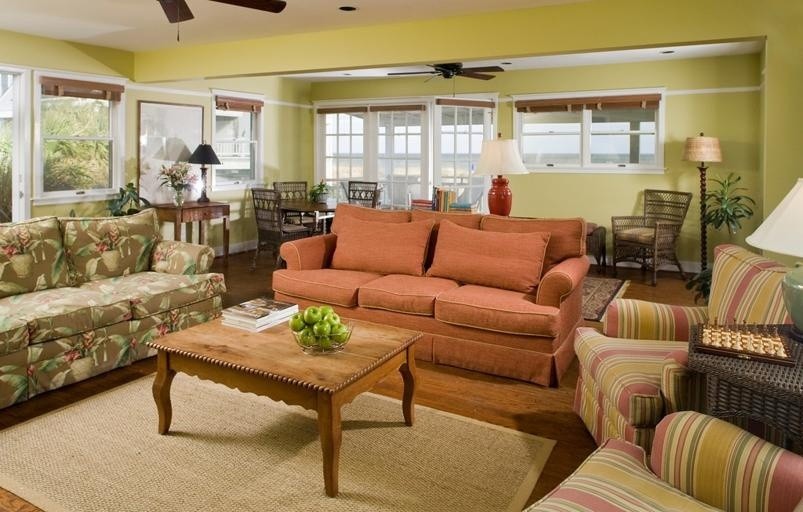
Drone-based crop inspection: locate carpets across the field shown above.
[0,370,576,510]
[581,278,632,327]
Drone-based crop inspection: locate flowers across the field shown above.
[156,165,199,204]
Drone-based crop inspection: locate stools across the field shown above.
[582,223,606,273]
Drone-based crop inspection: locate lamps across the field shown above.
[190,142,223,202]
[741,176,801,340]
[473,133,529,217]
[679,130,725,299]
[153,137,194,167]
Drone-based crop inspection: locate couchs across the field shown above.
[269,203,588,389]
[0,207,228,412]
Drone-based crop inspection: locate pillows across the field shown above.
[428,220,552,295]
[328,215,436,276]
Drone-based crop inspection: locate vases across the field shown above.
[172,191,184,207]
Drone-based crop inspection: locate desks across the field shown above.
[146,201,230,267]
[686,318,802,456]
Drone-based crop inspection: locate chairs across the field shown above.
[569,245,795,451]
[611,188,691,286]
[248,177,380,271]
[524,411,802,511]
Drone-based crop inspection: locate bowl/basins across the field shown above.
[290,317,354,355]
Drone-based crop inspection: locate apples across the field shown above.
[289,305,348,350]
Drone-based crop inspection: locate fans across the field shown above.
[156,0,286,25]
[387,63,504,83]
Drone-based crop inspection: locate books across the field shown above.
[221,297,299,333]
[411,186,476,214]
